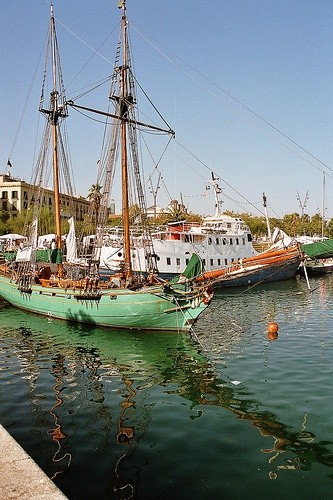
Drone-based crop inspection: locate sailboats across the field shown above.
[0,0,212,332]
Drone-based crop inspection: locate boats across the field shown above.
[84,175,333,288]
